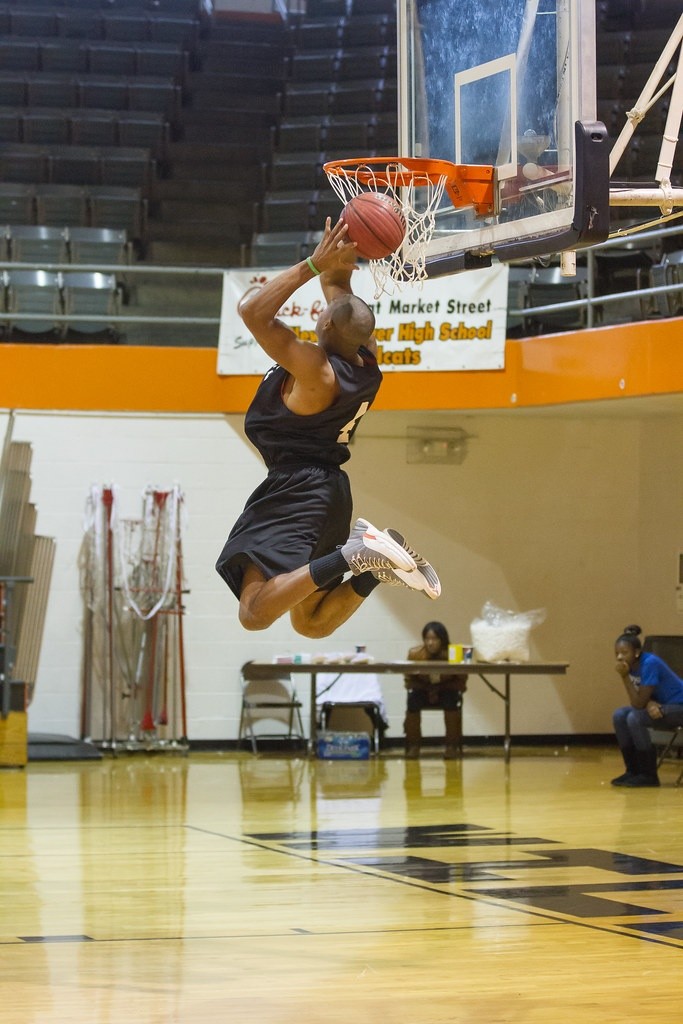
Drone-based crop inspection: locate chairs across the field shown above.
[312,651,389,759]
[0,0,683,346]
[236,659,307,755]
[642,634,683,782]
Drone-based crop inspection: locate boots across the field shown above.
[611,746,637,786]
[623,748,660,789]
[403,708,420,759]
[444,708,459,758]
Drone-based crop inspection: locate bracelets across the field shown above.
[306,256,321,275]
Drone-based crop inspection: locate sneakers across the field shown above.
[337,517,418,576]
[371,528,442,601]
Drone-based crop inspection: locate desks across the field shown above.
[252,661,570,763]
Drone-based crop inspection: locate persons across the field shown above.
[403,620,469,761]
[214,216,441,640]
[611,624,683,788]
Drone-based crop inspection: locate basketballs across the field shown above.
[338,191,408,260]
